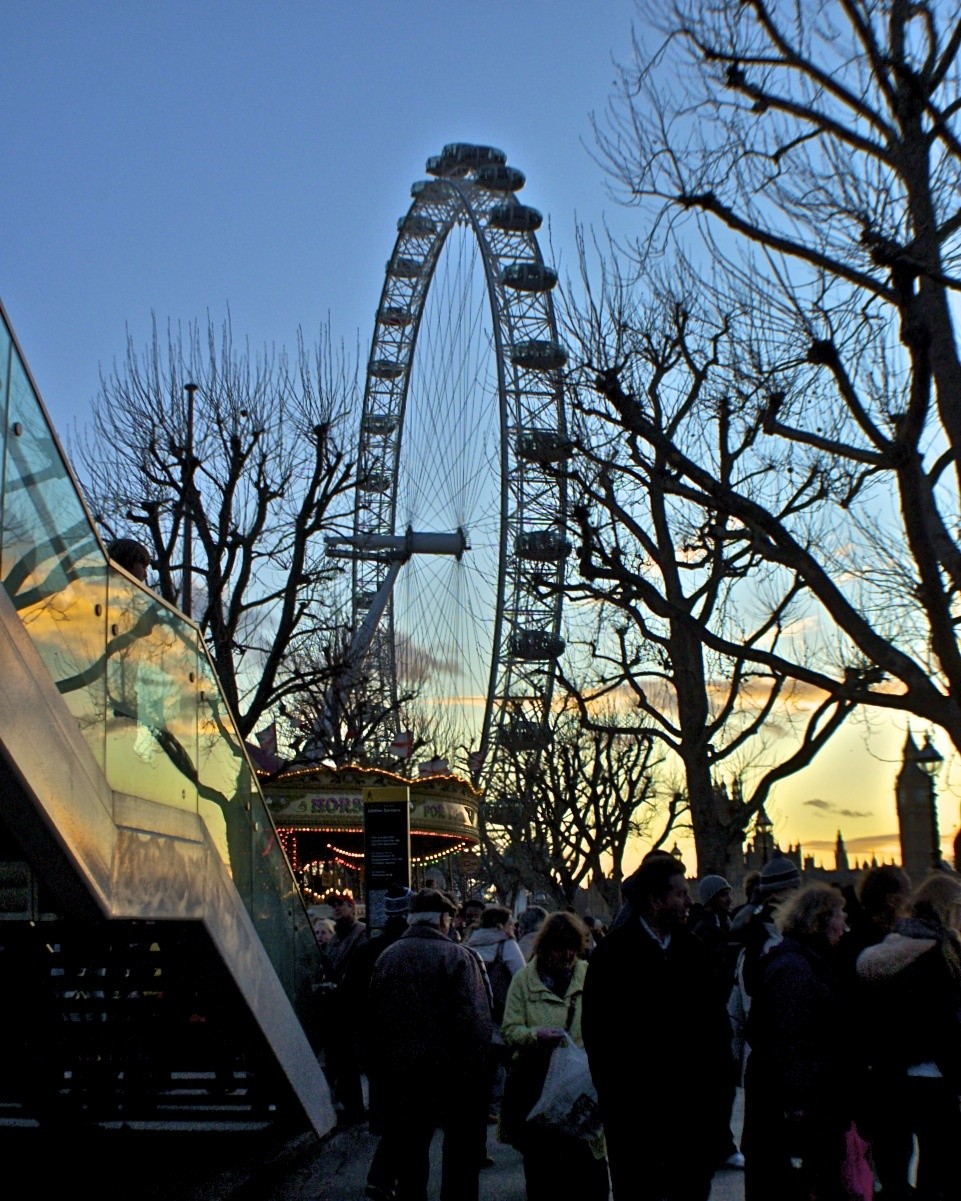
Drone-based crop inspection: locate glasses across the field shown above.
[332,901,347,908]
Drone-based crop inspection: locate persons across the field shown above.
[314,849,961,1201]
[107,539,151,582]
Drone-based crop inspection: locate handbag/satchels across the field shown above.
[526,1029,607,1160]
[844,1120,874,1201]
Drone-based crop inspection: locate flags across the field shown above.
[255,722,278,760]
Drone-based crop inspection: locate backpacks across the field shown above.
[482,942,513,1026]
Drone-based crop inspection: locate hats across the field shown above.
[383,887,415,915]
[758,852,800,892]
[324,893,354,904]
[698,875,730,905]
[519,905,548,932]
[410,888,457,913]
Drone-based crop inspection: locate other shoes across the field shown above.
[484,1156,494,1168]
[364,1184,397,1201]
[722,1152,745,1169]
